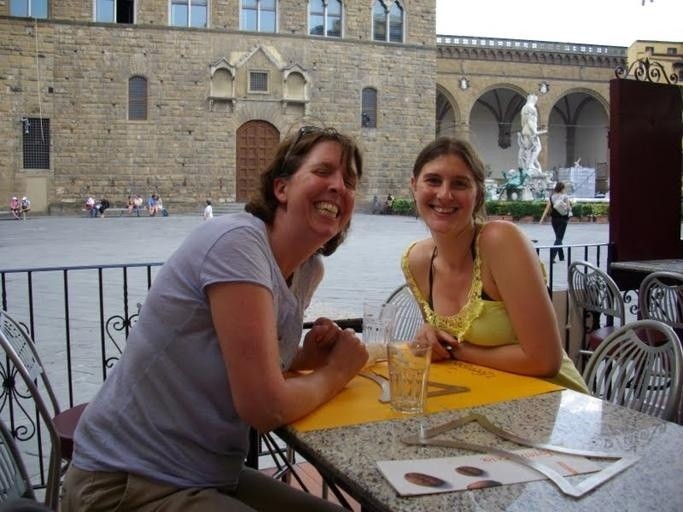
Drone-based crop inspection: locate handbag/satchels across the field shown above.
[552,200,568,216]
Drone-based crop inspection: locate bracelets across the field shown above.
[445,343,456,360]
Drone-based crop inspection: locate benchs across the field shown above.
[82,208,168,217]
[0,210,25,220]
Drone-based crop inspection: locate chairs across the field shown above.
[568,259,683,422]
[0,308,89,512]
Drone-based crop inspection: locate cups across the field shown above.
[358,299,399,360]
[386,336,436,414]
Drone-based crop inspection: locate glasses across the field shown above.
[287,126,318,166]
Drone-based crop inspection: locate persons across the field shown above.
[537,181,572,263]
[397,134,590,394]
[515,92,548,173]
[18,197,31,220]
[125,193,163,216]
[97,195,109,218]
[202,198,212,221]
[500,169,522,203]
[85,195,98,217]
[371,193,395,214]
[10,196,20,220]
[62,112,371,512]
